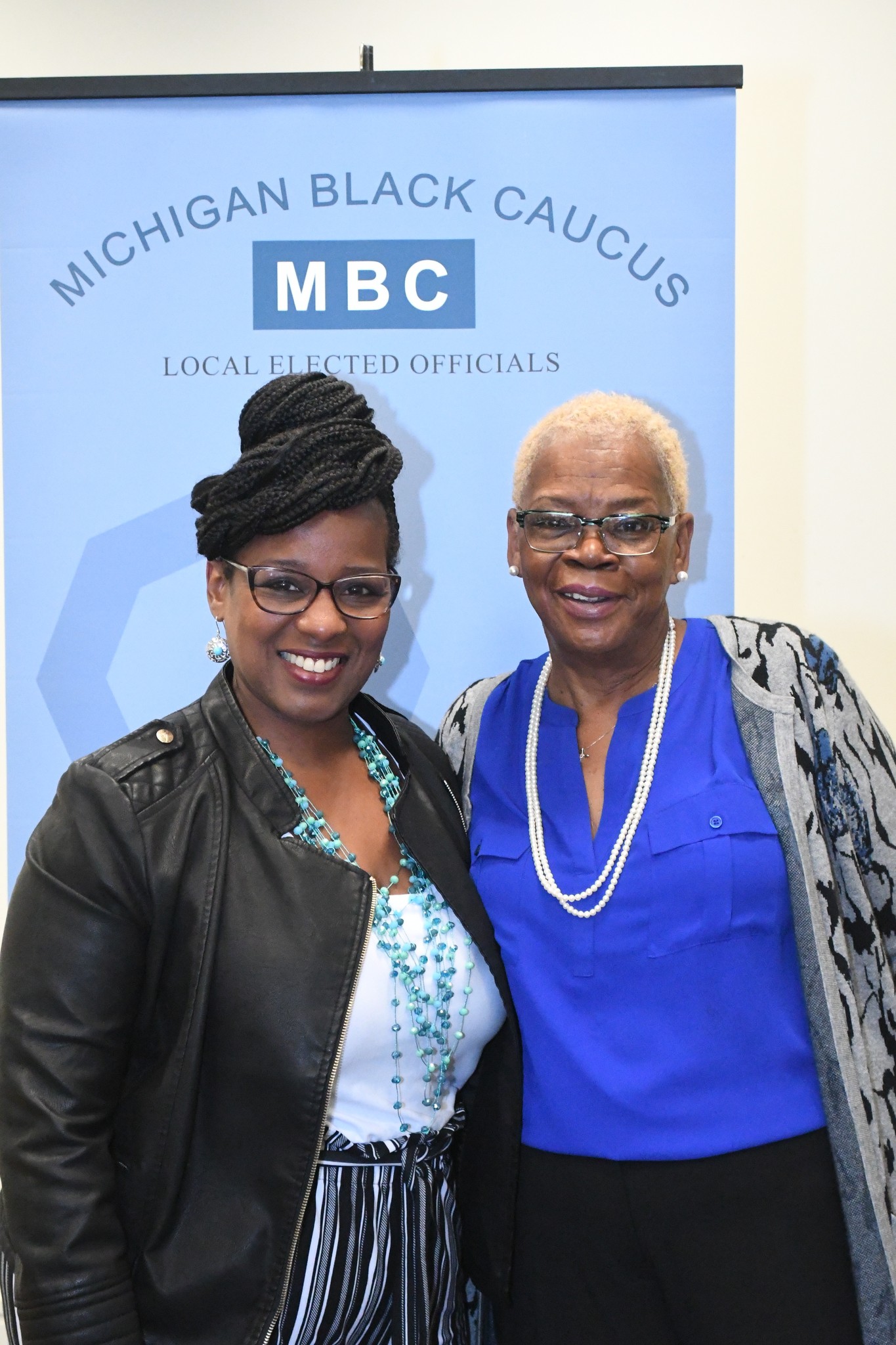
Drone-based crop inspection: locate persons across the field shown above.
[1,366,521,1345]
[439,386,896,1345]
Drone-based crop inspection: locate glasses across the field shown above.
[214,554,402,619]
[516,506,681,556]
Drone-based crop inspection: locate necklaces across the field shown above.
[573,706,619,766]
[250,707,476,1140]
[518,615,683,918]
